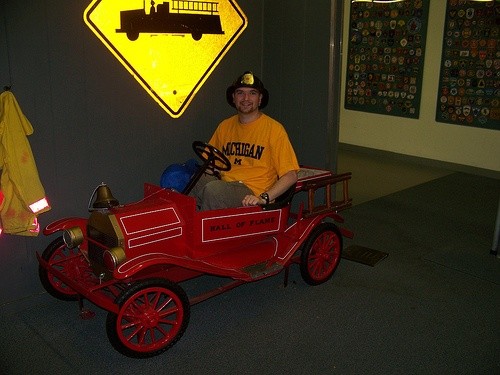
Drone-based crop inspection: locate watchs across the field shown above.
[260,192,270,205]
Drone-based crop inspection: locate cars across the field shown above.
[37,139,351,359]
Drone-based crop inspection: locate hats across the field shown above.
[226,71,270,110]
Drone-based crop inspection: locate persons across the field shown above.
[182,71,300,210]
